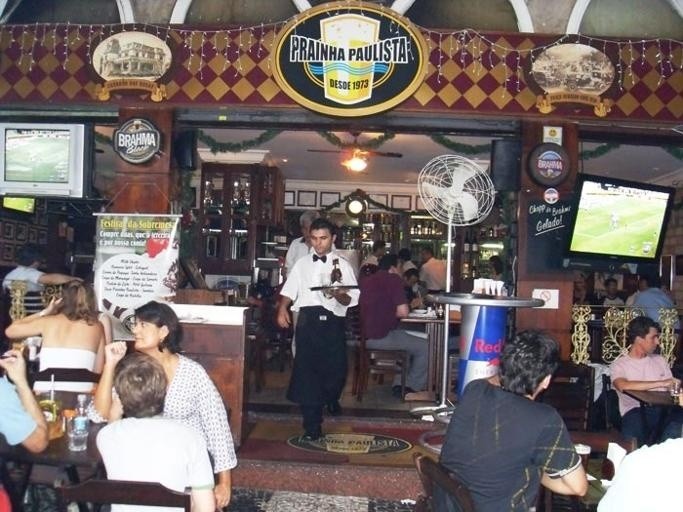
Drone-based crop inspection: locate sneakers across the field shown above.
[392,385,415,397]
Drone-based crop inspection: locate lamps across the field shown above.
[342,149,369,173]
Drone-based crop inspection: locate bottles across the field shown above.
[342,214,394,262]
[332,258,342,285]
[437,304,444,317]
[70,394,90,451]
[410,221,444,239]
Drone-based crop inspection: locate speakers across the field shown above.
[491,140,521,191]
[171,129,198,169]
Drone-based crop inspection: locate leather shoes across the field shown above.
[327,400,342,415]
[304,430,320,440]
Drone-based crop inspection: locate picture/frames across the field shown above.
[319,192,340,206]
[297,190,316,207]
[391,195,410,210]
[368,194,388,209]
[416,195,436,211]
[284,191,295,206]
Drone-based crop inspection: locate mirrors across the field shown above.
[345,196,366,218]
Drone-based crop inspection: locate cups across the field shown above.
[574,444,591,469]
[672,379,680,394]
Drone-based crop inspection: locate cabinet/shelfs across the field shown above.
[285,209,453,263]
[200,163,285,274]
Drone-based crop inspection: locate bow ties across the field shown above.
[313,254,328,263]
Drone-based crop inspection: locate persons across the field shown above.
[274,218,363,445]
[0,242,86,320]
[357,240,446,397]
[4,279,108,511]
[96,348,219,512]
[0,348,50,511]
[92,300,238,511]
[435,327,587,512]
[281,211,322,371]
[245,266,289,368]
[68,281,112,347]
[598,269,680,330]
[489,255,505,281]
[594,437,682,511]
[611,317,682,444]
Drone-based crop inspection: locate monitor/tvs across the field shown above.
[0,116,94,200]
[565,172,676,264]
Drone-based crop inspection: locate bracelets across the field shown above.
[38,309,46,319]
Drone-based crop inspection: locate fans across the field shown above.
[409,154,496,415]
